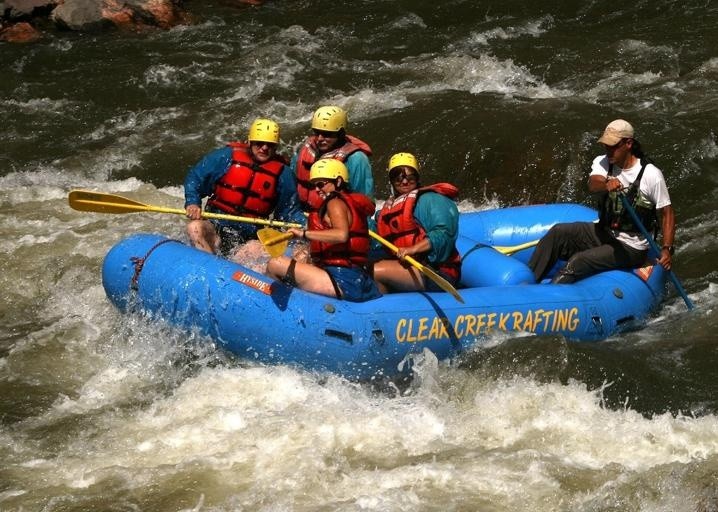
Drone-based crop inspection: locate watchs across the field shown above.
[662,246,674,256]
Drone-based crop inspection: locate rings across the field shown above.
[400,253,405,257]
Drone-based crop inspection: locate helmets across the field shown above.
[248,118,280,148]
[388,152,420,176]
[312,105,347,134]
[309,159,349,184]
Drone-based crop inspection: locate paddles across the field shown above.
[492,218,602,257]
[255,223,294,259]
[367,226,466,304]
[69,190,303,231]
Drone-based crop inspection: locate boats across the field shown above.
[51,189,671,391]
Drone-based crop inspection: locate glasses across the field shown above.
[393,174,417,184]
[311,180,332,190]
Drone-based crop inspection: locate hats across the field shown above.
[598,119,635,146]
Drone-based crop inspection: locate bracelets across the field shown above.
[303,230,307,241]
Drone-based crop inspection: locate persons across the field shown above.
[529,118,676,284]
[180,116,308,276]
[269,103,375,256]
[372,153,462,293]
[266,159,383,303]
